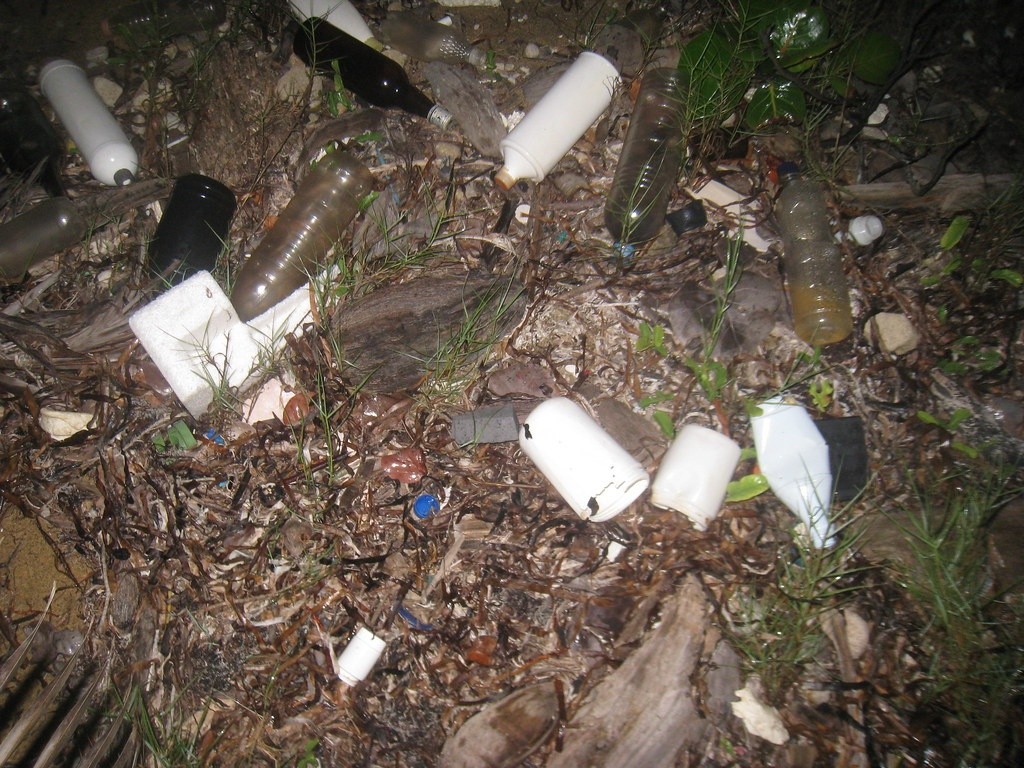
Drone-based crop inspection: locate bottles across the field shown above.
[773,161,854,345]
[375,11,489,68]
[230,150,374,323]
[101,0,229,41]
[286,0,409,69]
[292,17,454,130]
[0,196,89,281]
[493,51,624,192]
[39,59,139,187]
[0,62,70,200]
[334,628,387,687]
[140,173,238,288]
[845,216,883,247]
[604,67,692,257]
